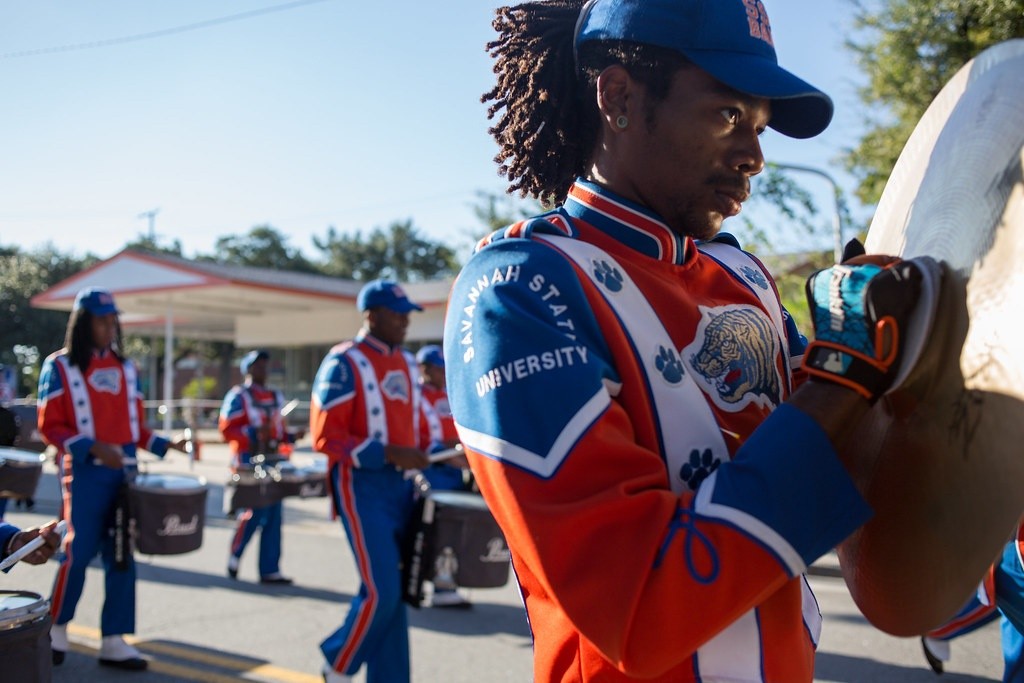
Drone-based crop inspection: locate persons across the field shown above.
[442,1,924,683]
[308,277,432,683]
[0,517,63,575]
[415,343,479,611]
[217,351,295,586]
[920,512,1024,683]
[828,126,1024,642]
[36,284,201,673]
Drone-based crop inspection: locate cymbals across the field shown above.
[834,37,1024,639]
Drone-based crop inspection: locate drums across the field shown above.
[0,447,42,496]
[232,455,328,508]
[0,590,53,683]
[423,490,511,589]
[122,472,209,557]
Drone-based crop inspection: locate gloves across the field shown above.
[801,238,923,409]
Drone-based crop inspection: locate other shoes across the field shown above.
[98,635,148,672]
[260,572,291,584]
[920,635,943,675]
[228,554,239,578]
[432,592,473,610]
[49,623,68,666]
[321,660,351,682]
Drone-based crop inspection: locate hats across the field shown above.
[357,279,424,314]
[240,350,270,375]
[573,0,834,139]
[74,287,124,317]
[416,345,445,367]
